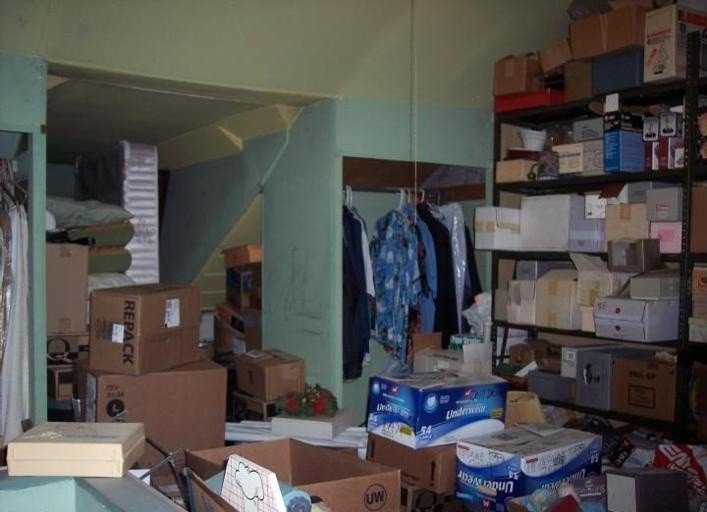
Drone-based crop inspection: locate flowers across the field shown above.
[276,382,339,418]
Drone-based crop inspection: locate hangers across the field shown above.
[344,183,352,210]
[394,188,405,210]
[0,162,30,213]
[418,187,425,203]
[405,187,411,203]
[425,186,440,205]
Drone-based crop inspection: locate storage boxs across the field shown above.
[570,139,606,178]
[84,359,228,468]
[560,61,592,105]
[570,179,683,271]
[495,121,546,183]
[408,327,483,373]
[236,347,304,399]
[593,299,678,344]
[493,53,543,96]
[472,205,520,251]
[184,436,401,511]
[367,370,508,450]
[519,194,568,253]
[603,93,644,175]
[631,274,681,300]
[611,355,675,424]
[367,433,457,495]
[535,254,610,330]
[593,47,643,97]
[45,244,90,336]
[492,257,535,326]
[577,269,615,305]
[574,349,611,411]
[47,365,77,405]
[688,177,706,345]
[538,39,572,73]
[568,4,644,60]
[494,92,564,114]
[271,408,345,439]
[497,339,575,403]
[7,422,146,479]
[213,244,261,364]
[605,467,689,511]
[579,305,597,334]
[455,422,603,512]
[643,4,707,85]
[88,284,200,376]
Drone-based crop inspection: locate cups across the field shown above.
[536,151,559,182]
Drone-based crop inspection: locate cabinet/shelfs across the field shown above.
[490,32,692,445]
